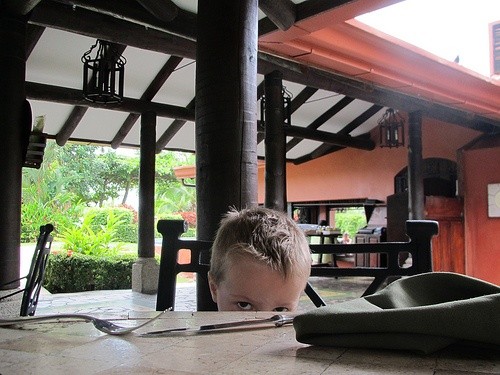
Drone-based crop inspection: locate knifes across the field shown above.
[141,314,312,339]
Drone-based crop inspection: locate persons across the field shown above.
[340,232,352,244]
[208,207,312,311]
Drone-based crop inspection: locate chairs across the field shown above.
[156,218,439,312]
[0,224,54,320]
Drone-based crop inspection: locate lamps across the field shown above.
[377,108,405,148]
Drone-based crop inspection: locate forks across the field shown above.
[0,305,174,334]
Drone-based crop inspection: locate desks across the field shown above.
[304,230,343,279]
[0,312,500,375]
